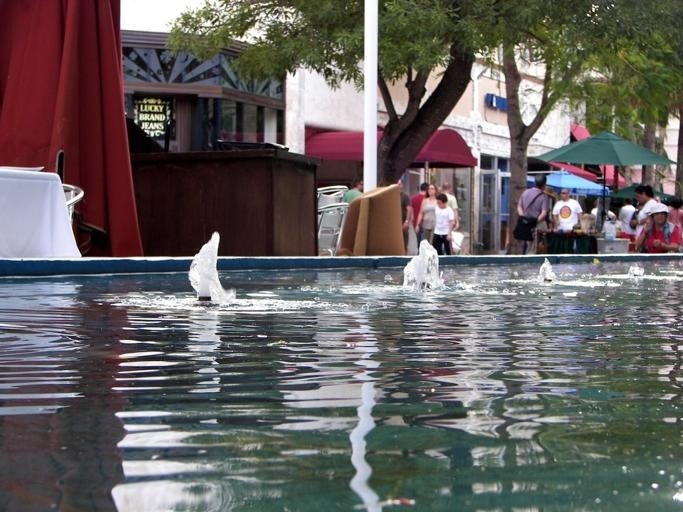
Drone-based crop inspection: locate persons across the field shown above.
[587,183,683,253]
[396,178,460,255]
[551,187,583,254]
[340,175,364,205]
[515,174,549,254]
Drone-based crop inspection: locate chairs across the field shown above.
[316,184,349,206]
[338,182,406,256]
[317,202,351,255]
[60,182,85,227]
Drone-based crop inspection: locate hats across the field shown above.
[649,203,670,215]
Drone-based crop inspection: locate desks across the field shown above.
[0,167,86,259]
[129,150,320,258]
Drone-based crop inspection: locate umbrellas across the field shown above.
[532,129,676,214]
[533,167,609,199]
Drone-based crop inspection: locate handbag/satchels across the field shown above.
[513,213,536,240]
